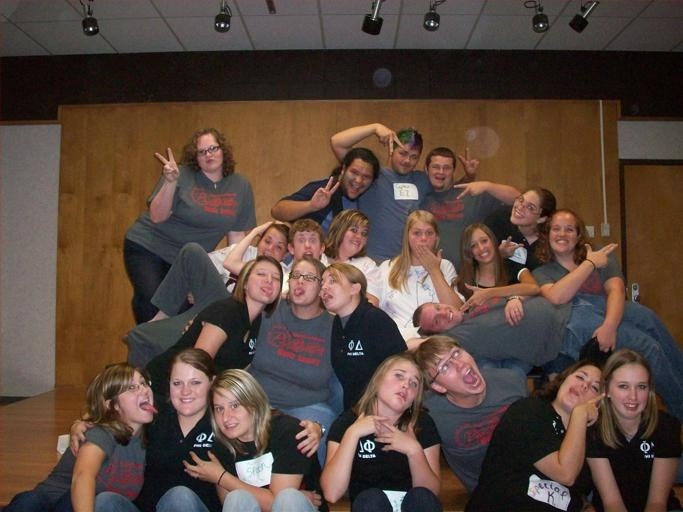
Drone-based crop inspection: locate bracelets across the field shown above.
[215,470,228,485]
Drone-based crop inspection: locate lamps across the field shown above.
[72,0,606,37]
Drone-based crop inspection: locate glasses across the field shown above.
[429,348,463,384]
[517,195,541,214]
[290,272,321,282]
[127,381,153,392]
[197,146,220,155]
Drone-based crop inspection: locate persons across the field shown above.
[412,332,530,494]
[122,127,255,328]
[121,186,683,471]
[180,368,329,512]
[2,362,156,510]
[68,346,327,512]
[460,361,605,511]
[585,349,682,511]
[271,122,522,274]
[319,353,443,511]
